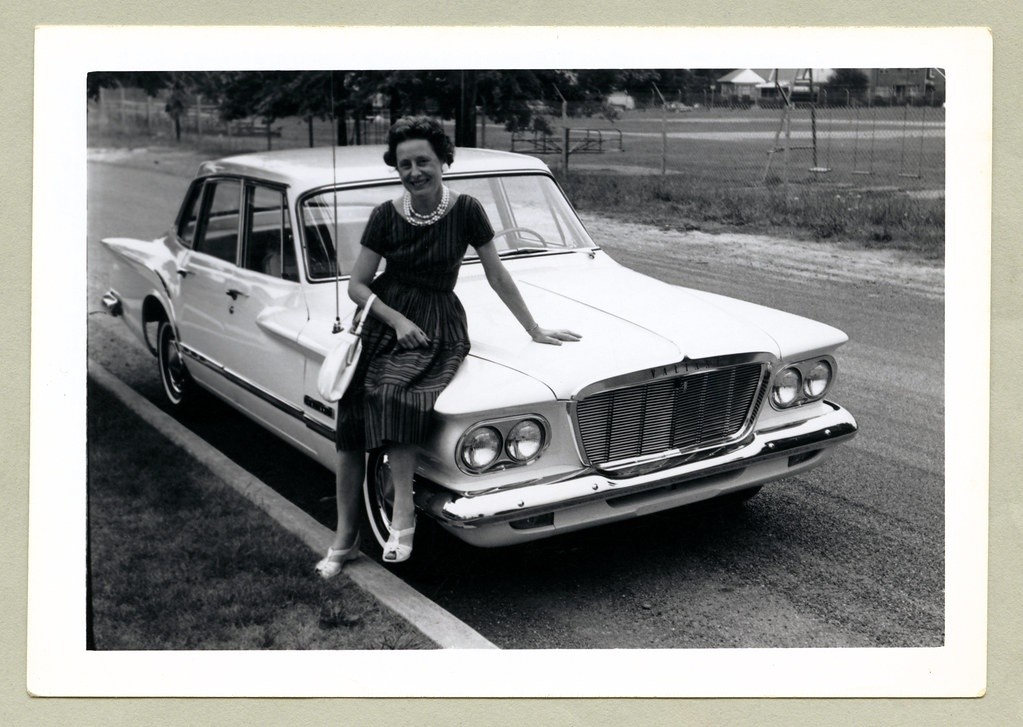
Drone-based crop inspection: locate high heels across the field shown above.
[314,529,362,579]
[382,516,415,563]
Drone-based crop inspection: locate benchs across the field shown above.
[202,220,385,282]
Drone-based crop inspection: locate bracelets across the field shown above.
[526,324,539,334]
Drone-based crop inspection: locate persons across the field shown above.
[314,114,583,579]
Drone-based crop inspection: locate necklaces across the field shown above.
[403,183,450,226]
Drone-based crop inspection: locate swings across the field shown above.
[897,68,930,179]
[807,68,835,174]
[851,68,880,176]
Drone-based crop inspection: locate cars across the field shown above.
[663,101,693,114]
[99,140,863,582]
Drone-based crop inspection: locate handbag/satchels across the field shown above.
[315,293,376,403]
